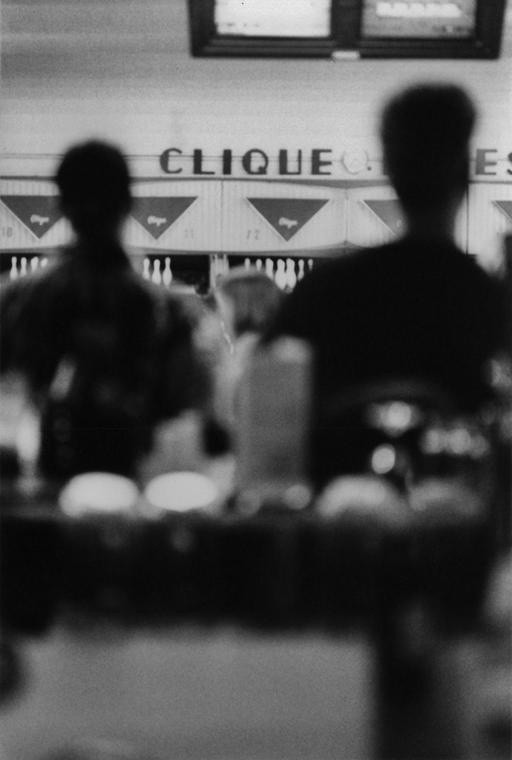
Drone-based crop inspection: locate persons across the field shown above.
[2,81,511,508]
